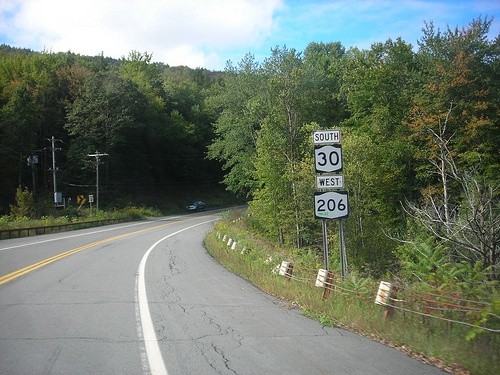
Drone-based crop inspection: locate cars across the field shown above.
[186,200,206,213]
